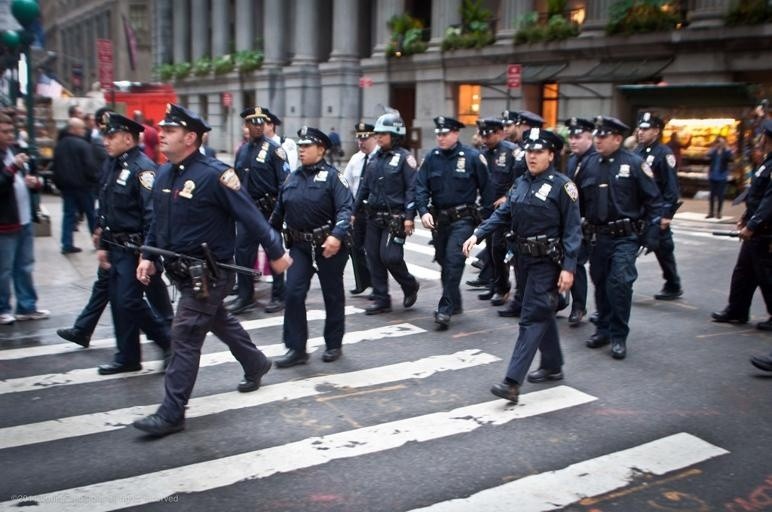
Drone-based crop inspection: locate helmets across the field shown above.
[373,111,407,135]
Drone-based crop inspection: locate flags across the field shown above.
[120,13,139,71]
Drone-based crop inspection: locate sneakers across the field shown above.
[757,316,772,332]
[134,414,185,435]
[711,307,749,324]
[1,313,15,324]
[61,246,82,254]
[750,354,771,371]
[15,309,50,320]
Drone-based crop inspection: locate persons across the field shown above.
[709,103,772,373]
[200,105,420,366]
[412,111,683,402]
[131,104,292,435]
[1,106,175,374]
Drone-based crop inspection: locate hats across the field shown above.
[433,116,466,134]
[296,126,333,146]
[521,128,563,151]
[355,122,378,140]
[564,117,596,136]
[500,111,520,126]
[512,111,547,128]
[593,116,630,138]
[638,114,664,130]
[476,119,504,137]
[99,103,282,136]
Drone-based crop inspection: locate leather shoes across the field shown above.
[274,349,308,368]
[655,285,683,299]
[58,328,89,347]
[265,300,286,313]
[366,304,392,315]
[98,362,142,375]
[491,379,519,402]
[527,367,563,382]
[237,358,272,390]
[403,281,419,307]
[436,260,627,360]
[229,283,240,295]
[224,297,256,314]
[322,347,341,363]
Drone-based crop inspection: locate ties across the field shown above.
[361,155,368,178]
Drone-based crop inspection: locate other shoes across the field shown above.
[350,285,368,294]
[367,292,375,301]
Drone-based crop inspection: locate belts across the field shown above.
[288,227,314,241]
[364,208,392,221]
[434,208,461,220]
[512,236,562,249]
[585,218,611,228]
